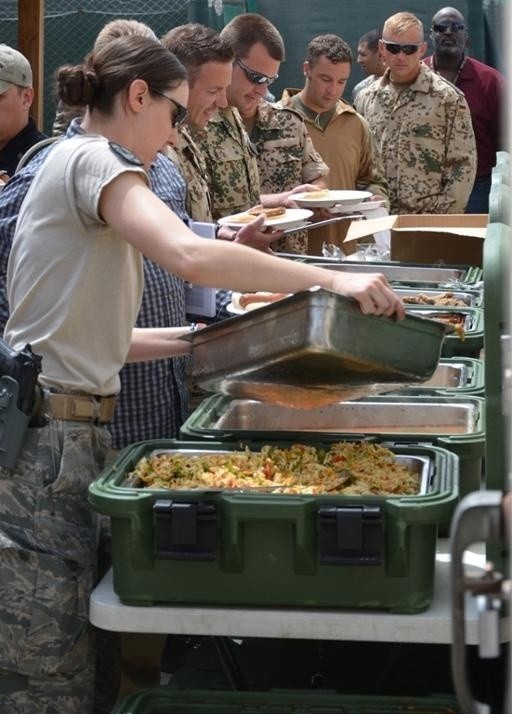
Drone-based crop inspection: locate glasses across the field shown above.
[381,37,424,55]
[433,23,465,33]
[150,87,189,131]
[235,59,279,85]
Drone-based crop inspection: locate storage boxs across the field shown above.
[340,203,493,265]
[83,250,492,614]
[112,683,463,713]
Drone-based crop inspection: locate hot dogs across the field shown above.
[232,291,288,312]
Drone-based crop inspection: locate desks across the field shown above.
[85,528,498,655]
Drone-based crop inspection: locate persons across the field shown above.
[1,6,512,448]
[2,34,406,713]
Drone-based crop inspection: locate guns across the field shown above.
[0,340,43,473]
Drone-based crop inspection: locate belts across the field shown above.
[47,393,118,424]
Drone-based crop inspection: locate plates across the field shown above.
[217,208,313,233]
[288,188,374,207]
[226,302,272,315]
[327,199,387,213]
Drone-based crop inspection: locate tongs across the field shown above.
[267,214,367,262]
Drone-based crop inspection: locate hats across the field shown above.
[0,43,33,96]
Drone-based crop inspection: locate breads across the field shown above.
[248,206,285,216]
[303,188,328,200]
[231,215,258,222]
